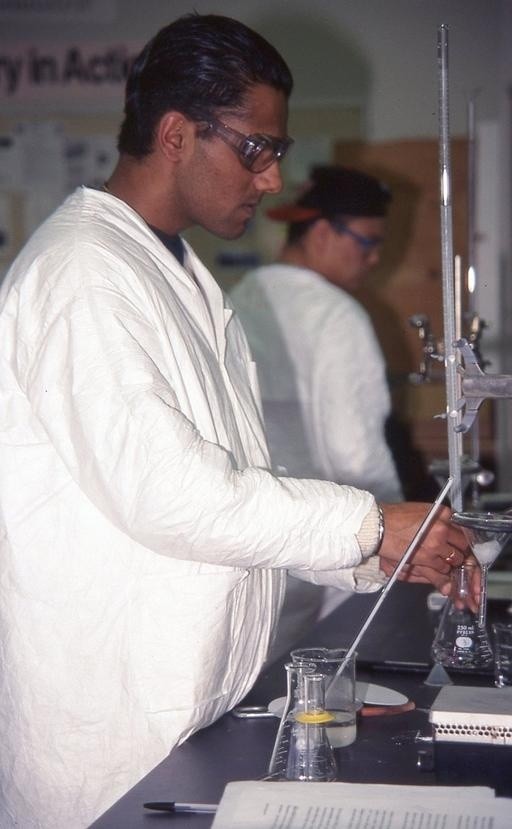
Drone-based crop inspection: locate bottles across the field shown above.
[430,564,495,669]
[269,662,337,781]
[304,674,325,782]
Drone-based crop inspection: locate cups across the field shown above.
[290,648,358,748]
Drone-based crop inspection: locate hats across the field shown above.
[262,161,393,224]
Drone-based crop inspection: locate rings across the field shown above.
[446,549,456,562]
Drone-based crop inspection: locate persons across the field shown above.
[229,160,406,673]
[1,8,483,829]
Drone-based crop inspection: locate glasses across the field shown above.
[190,101,296,173]
[332,215,390,249]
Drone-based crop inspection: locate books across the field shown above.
[210,778,512,828]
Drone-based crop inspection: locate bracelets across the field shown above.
[372,501,385,558]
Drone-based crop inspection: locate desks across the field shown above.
[87,582,512,829]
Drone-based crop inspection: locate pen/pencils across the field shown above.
[141,800,217,816]
